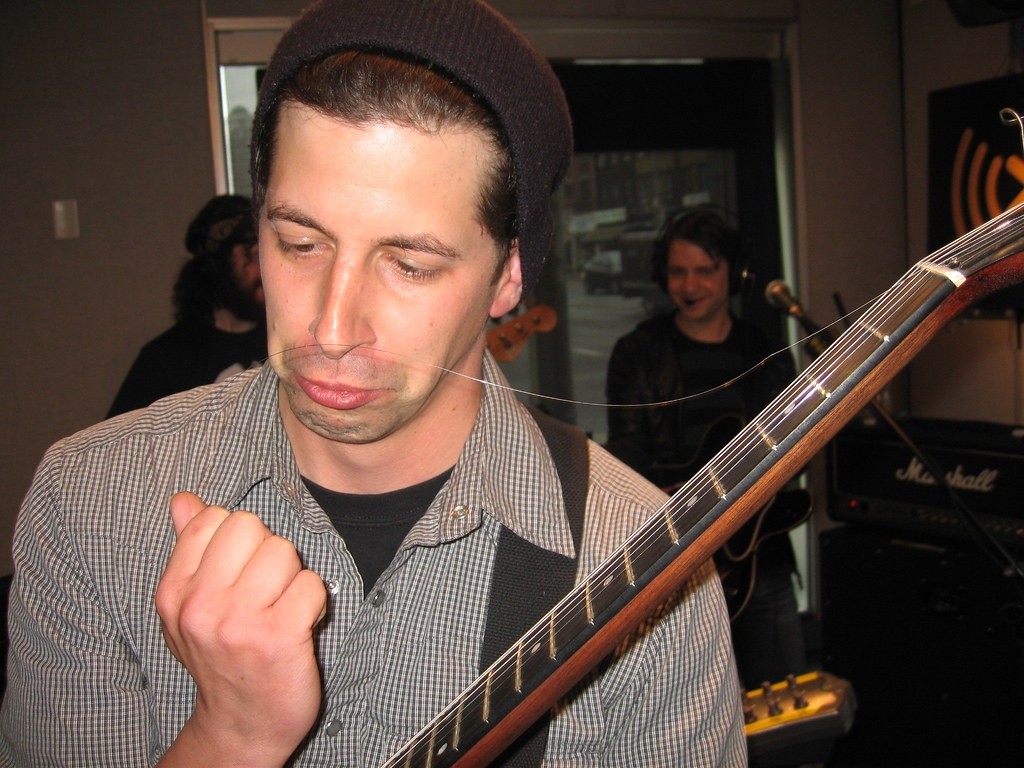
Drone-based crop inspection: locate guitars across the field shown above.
[738,669,858,755]
[358,199,1022,768]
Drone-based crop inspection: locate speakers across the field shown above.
[816,522,1024,768]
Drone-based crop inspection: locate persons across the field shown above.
[104,195,268,420]
[0,1,750,767]
[602,204,815,693]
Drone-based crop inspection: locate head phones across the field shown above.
[648,207,755,297]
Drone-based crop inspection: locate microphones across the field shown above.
[683,296,704,307]
[764,280,806,319]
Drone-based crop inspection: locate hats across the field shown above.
[185,193,258,253]
[251,0,572,291]
